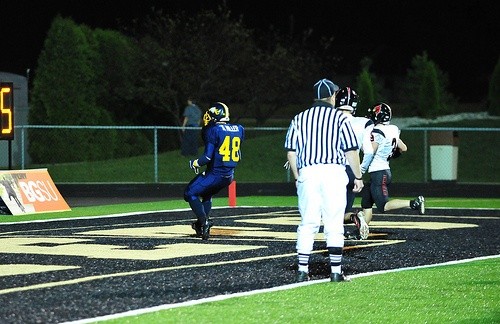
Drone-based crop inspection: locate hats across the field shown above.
[314,78,339,99]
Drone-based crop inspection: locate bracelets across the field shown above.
[356,178,362,180]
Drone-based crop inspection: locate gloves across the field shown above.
[352,178,364,193]
[189,159,200,169]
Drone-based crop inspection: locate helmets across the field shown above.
[202,102,230,126]
[371,103,392,123]
[335,87,359,110]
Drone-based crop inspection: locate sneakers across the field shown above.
[359,210,369,240]
[192,223,202,236]
[418,195,425,215]
[201,218,214,240]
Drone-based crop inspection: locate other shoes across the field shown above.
[350,213,361,229]
[295,271,312,283]
[330,271,351,283]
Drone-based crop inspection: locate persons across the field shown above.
[361,102,425,224]
[284,78,364,283]
[184,102,244,239]
[333,86,375,240]
[182,95,202,156]
